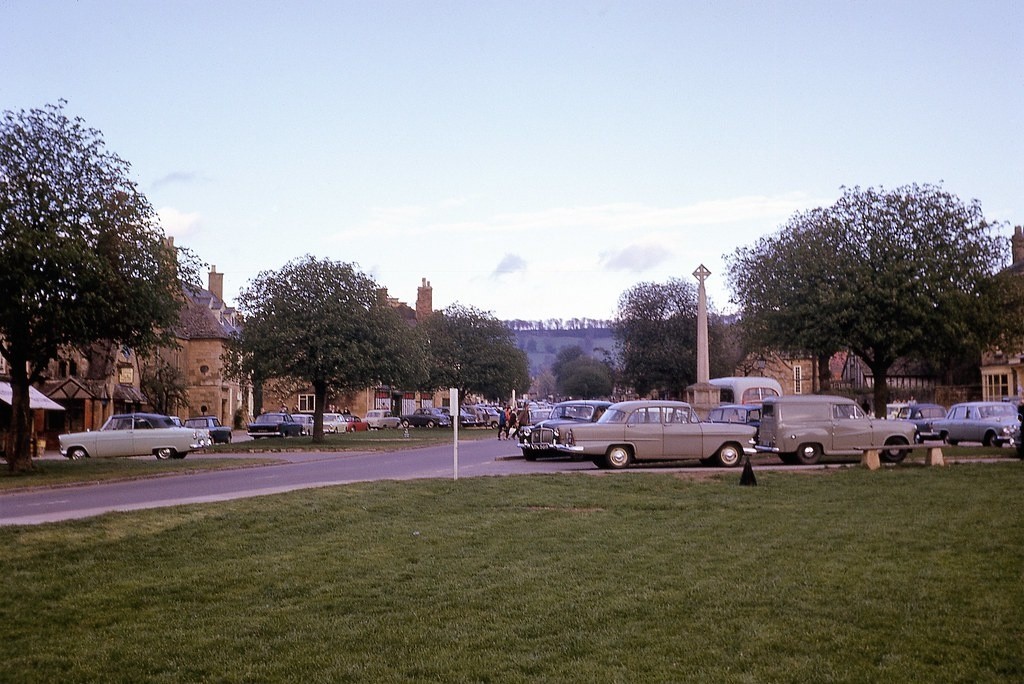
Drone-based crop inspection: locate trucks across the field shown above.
[708,376,783,406]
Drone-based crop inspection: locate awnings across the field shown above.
[113,383,149,401]
[0,381,65,410]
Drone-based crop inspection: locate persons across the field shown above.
[497,403,530,441]
[278,402,389,415]
[861,396,918,415]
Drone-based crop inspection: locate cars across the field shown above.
[519,400,612,463]
[399,406,451,429]
[931,402,1024,448]
[341,414,371,433]
[365,410,402,431]
[59,413,208,463]
[291,414,314,436]
[757,394,919,464]
[552,401,758,468]
[437,406,476,428]
[871,400,952,443]
[184,416,232,444]
[461,399,556,428]
[246,413,303,440]
[704,404,771,449]
[322,413,347,435]
